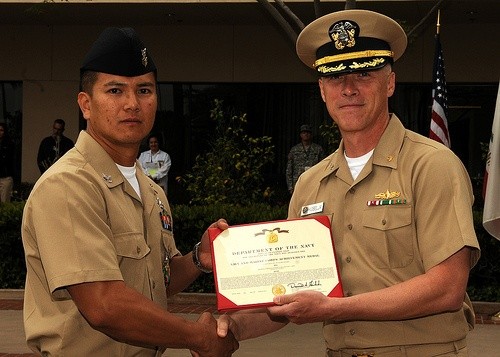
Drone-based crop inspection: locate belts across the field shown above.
[326,337,467,357]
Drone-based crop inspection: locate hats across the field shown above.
[296,10,407,76]
[300,125,312,132]
[80,27,157,77]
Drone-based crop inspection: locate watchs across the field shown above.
[192,242,213,273]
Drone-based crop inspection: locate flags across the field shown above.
[483,83,500,241]
[430,35,451,149]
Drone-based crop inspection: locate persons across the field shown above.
[286,125,327,194]
[21,27,239,357]
[37,119,76,175]
[190,9,481,357]
[138,136,171,199]
